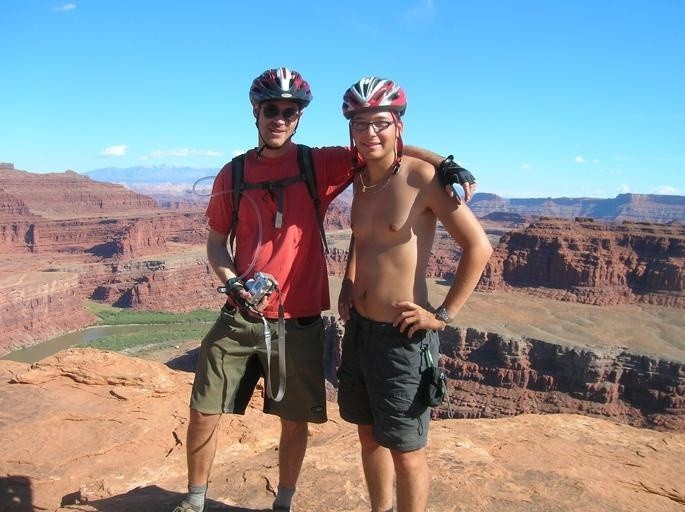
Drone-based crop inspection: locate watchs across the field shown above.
[436,305,453,324]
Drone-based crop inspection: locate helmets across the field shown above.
[248,67,313,112]
[342,77,406,120]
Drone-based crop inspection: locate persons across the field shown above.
[172,67,477,511]
[338,77,495,512]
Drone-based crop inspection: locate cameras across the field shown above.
[245,272,279,314]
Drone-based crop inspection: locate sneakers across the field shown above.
[168,498,209,512]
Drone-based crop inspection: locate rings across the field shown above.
[340,302,345,307]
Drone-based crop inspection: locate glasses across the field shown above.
[258,102,300,123]
[349,120,395,131]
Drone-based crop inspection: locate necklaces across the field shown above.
[359,169,393,192]
